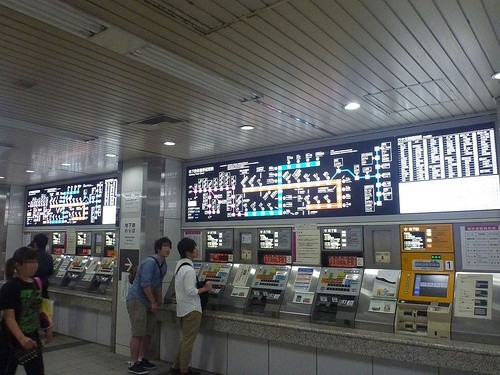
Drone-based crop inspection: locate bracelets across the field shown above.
[44,325,51,331]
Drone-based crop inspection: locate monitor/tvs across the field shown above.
[412,273,449,298]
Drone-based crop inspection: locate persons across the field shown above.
[28,234,54,327]
[170,237,212,375]
[126,237,171,375]
[0,247,52,375]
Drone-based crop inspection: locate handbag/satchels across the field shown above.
[176,262,209,312]
[34,276,55,330]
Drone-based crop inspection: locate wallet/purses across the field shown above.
[12,346,40,365]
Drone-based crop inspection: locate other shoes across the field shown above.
[169,368,180,375]
[180,369,201,375]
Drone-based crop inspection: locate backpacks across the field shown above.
[128,255,165,285]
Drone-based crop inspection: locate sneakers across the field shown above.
[127,360,150,374]
[137,357,157,370]
